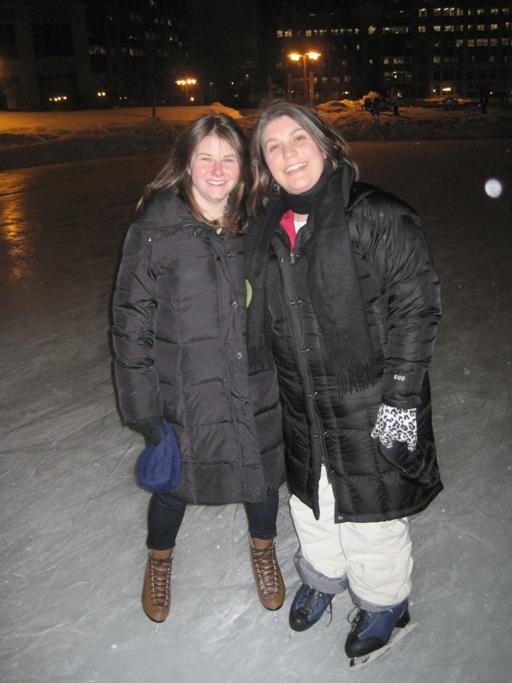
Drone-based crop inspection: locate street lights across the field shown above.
[286,50,322,103]
[175,79,197,105]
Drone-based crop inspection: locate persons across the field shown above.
[107,109,299,619]
[240,96,444,665]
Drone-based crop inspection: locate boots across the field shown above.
[290,585,334,632]
[249,536,285,613]
[141,547,174,623]
[345,598,410,659]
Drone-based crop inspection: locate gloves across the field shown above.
[370,404,418,452]
